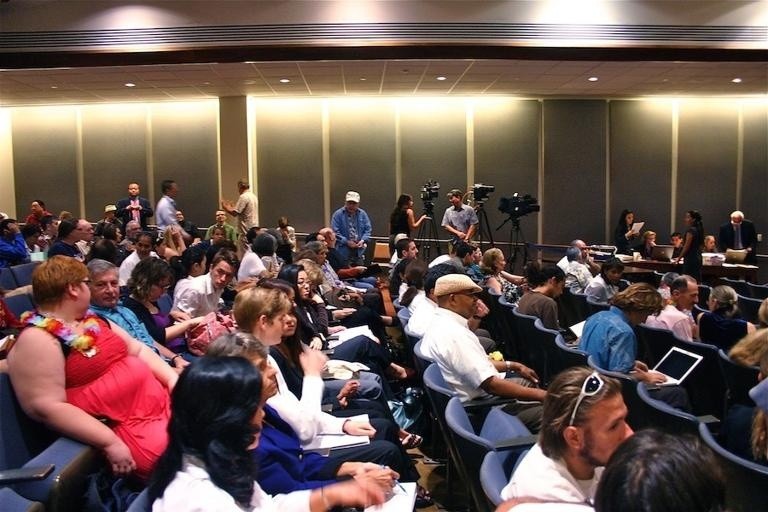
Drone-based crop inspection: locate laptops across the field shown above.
[645,345,704,387]
[650,244,675,260]
[726,250,747,263]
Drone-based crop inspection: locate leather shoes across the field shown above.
[468,394,498,405]
[391,368,416,380]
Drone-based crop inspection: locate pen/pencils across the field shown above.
[380,463,410,497]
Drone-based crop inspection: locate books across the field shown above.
[328,324,379,349]
[300,414,370,457]
[569,320,586,338]
[322,358,370,380]
[726,248,747,261]
[362,482,418,511]
[631,222,644,234]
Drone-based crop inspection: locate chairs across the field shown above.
[1,370,93,512]
[10,261,41,288]
[3,294,33,327]
[389,271,768,512]
[0,265,17,295]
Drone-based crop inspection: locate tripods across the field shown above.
[509,228,531,272]
[417,212,441,263]
[476,202,494,252]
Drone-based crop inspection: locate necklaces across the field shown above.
[74,342,100,358]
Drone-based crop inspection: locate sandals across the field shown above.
[414,482,430,509]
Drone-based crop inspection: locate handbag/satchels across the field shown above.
[183,312,231,357]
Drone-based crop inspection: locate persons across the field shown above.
[557,239,601,278]
[673,209,706,287]
[114,182,153,238]
[718,211,758,284]
[730,298,768,366]
[203,224,226,247]
[697,285,757,356]
[225,240,236,285]
[123,255,205,362]
[669,232,684,258]
[277,263,416,401]
[95,239,117,262]
[102,223,122,248]
[440,189,480,244]
[478,248,517,302]
[6,254,179,481]
[154,180,192,239]
[169,246,207,298]
[0,218,27,268]
[578,282,667,384]
[420,273,548,435]
[148,231,168,262]
[118,231,162,287]
[407,263,496,353]
[269,312,424,448]
[86,258,191,376]
[595,430,727,512]
[94,205,122,236]
[718,353,768,467]
[294,258,402,343]
[330,190,372,266]
[318,228,376,291]
[614,209,642,255]
[450,241,484,279]
[168,250,241,323]
[305,233,386,315]
[233,287,434,506]
[656,272,698,339]
[276,215,297,253]
[652,275,699,341]
[638,231,657,259]
[389,239,419,302]
[145,355,383,512]
[300,241,381,312]
[390,233,408,267]
[238,233,278,282]
[246,226,281,273]
[48,219,88,264]
[496,366,633,512]
[205,332,401,499]
[398,260,429,316]
[26,200,53,226]
[163,224,186,251]
[61,242,86,262]
[517,259,567,330]
[563,246,594,295]
[209,244,238,301]
[176,208,202,239]
[20,224,44,252]
[703,235,718,254]
[78,219,95,256]
[221,176,259,246]
[584,258,631,306]
[388,194,433,257]
[257,277,394,421]
[267,228,292,264]
[205,209,237,244]
[468,243,484,266]
[37,215,59,249]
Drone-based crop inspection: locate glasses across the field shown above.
[568,369,604,427]
[731,220,742,225]
[80,279,92,287]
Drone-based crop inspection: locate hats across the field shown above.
[446,188,464,198]
[433,273,482,299]
[345,191,362,206]
[104,204,118,213]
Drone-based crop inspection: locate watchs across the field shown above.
[473,314,481,319]
[172,355,182,363]
[506,361,511,370]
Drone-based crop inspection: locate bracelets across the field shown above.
[321,484,329,510]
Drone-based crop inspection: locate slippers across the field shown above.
[396,429,423,447]
